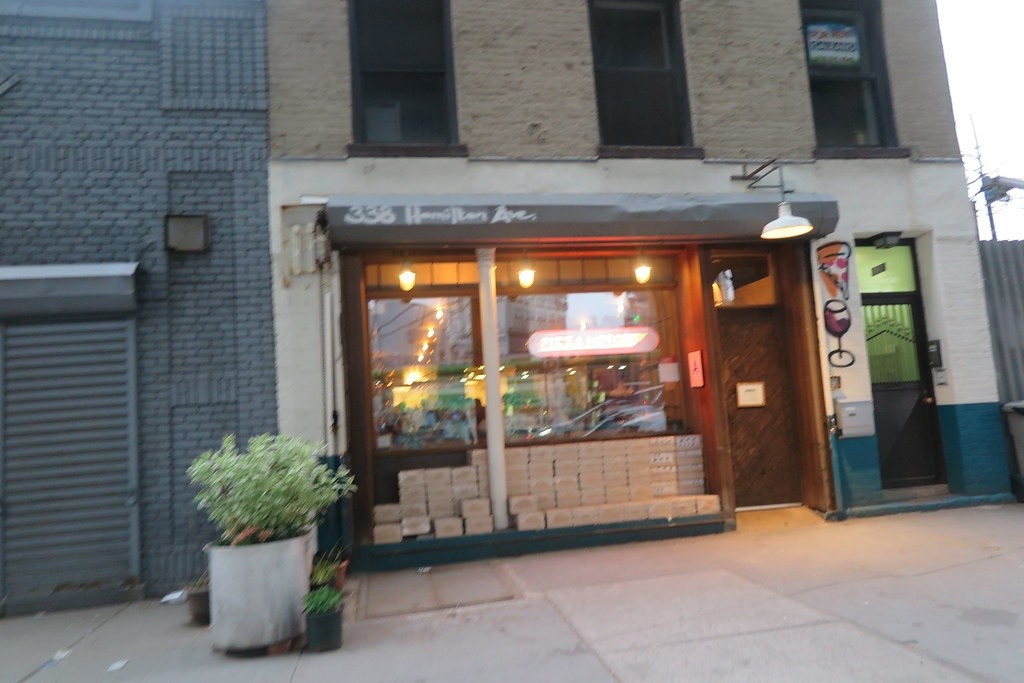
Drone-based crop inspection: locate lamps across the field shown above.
[871,231,902,249]
[633,246,651,284]
[396,251,418,291]
[517,258,536,289]
[749,164,814,240]
[167,212,210,252]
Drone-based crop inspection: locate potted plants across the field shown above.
[189,430,360,655]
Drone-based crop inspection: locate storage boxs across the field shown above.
[372,434,720,546]
